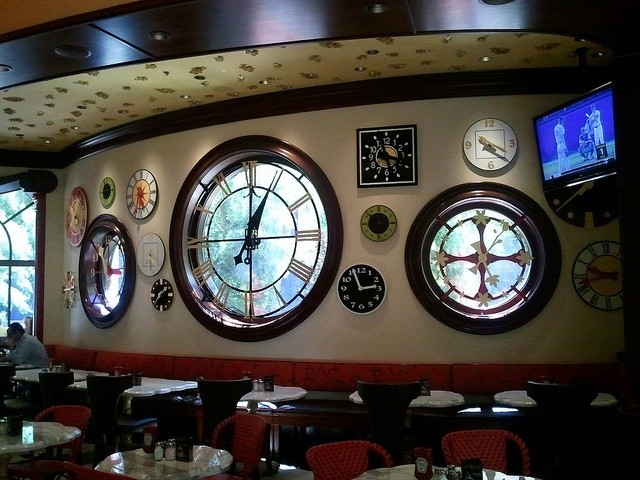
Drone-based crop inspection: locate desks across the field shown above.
[0,360,34,369]
[349,386,464,414]
[351,463,545,480]
[10,366,109,396]
[95,440,314,480]
[492,387,617,410]
[0,420,81,479]
[175,382,307,411]
[66,374,198,418]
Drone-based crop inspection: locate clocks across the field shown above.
[168,135,344,343]
[61,269,75,309]
[66,187,88,247]
[571,240,623,311]
[461,118,517,171]
[404,183,562,335]
[136,233,166,276]
[356,122,419,188]
[545,158,622,230]
[78,213,136,329]
[98,177,115,207]
[336,264,386,316]
[359,204,398,243]
[150,278,174,312]
[125,168,158,220]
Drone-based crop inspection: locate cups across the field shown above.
[22,419,33,444]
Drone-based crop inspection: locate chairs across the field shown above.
[37,372,74,399]
[355,381,429,432]
[33,405,92,465]
[0,365,30,413]
[85,375,158,452]
[441,430,531,478]
[27,459,138,480]
[306,441,393,480]
[526,381,598,429]
[211,413,266,475]
[197,376,271,446]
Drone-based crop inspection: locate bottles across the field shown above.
[132,370,141,386]
[165,443,175,461]
[161,440,166,458]
[115,366,122,377]
[48,358,54,370]
[258,381,264,390]
[155,443,163,462]
[62,361,70,372]
[168,439,176,448]
[254,380,258,392]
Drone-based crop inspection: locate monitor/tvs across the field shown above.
[531,81,618,194]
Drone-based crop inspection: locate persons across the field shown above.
[585,103,607,148]
[0,322,50,367]
[578,127,595,162]
[553,114,571,174]
[585,118,595,143]
